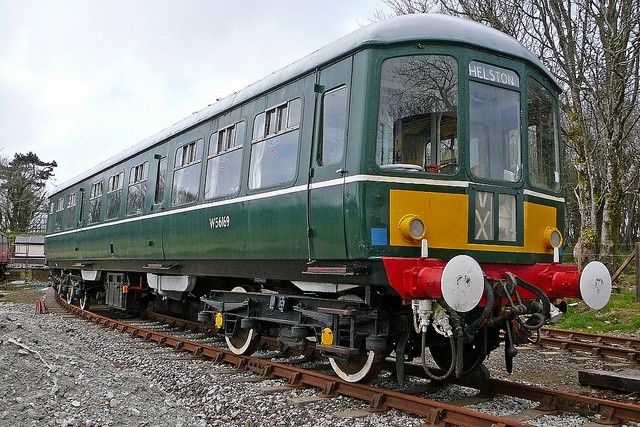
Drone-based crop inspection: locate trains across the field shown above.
[44,12,613,385]
